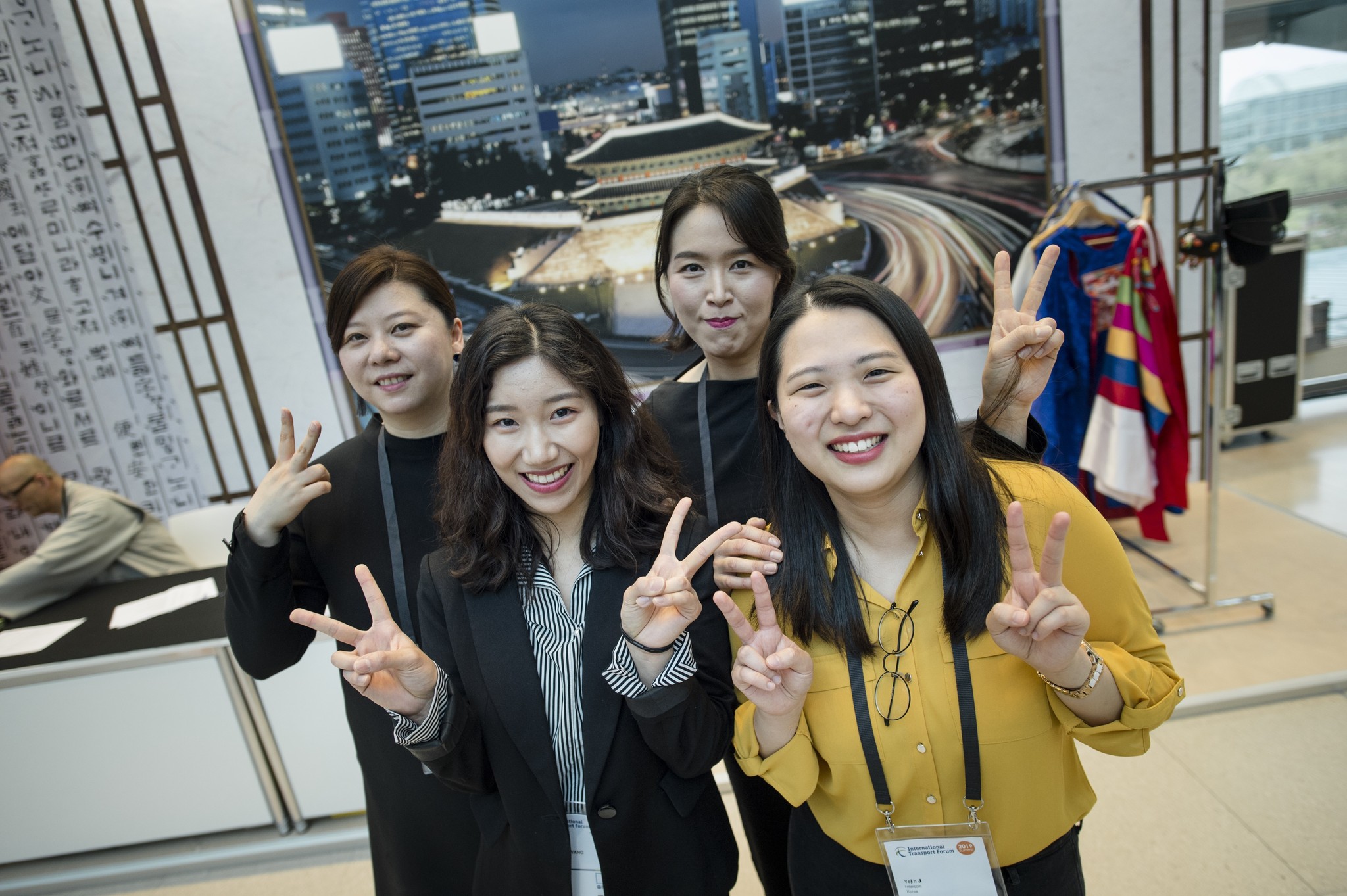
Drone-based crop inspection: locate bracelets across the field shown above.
[619,622,676,654]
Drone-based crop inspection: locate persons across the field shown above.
[698,273,1189,896]
[288,302,744,896]
[0,454,199,620]
[219,241,514,896]
[622,164,1066,896]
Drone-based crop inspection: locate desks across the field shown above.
[0,565,370,866]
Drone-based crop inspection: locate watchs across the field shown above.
[1035,637,1105,699]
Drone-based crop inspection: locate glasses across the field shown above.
[874,601,919,727]
[4,477,34,500]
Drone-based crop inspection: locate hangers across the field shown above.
[1022,171,1154,248]
